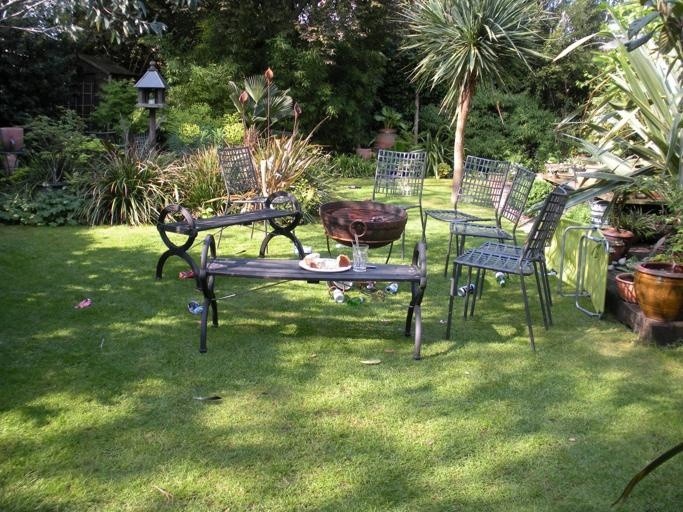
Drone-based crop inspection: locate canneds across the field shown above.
[187,301,202,315]
[384,282,399,294]
[332,288,345,303]
[74,298,93,310]
[457,285,478,300]
[179,270,195,280]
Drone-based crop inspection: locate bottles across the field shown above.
[348,297,365,307]
[496,271,506,288]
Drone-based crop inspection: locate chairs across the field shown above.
[421,149,571,333]
[413,147,569,351]
[215,146,303,254]
[370,145,430,262]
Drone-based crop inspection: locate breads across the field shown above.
[304,253,320,269]
[336,255,350,269]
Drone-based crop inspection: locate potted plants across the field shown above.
[575,157,682,326]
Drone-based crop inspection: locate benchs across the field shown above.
[195,232,430,362]
[155,190,309,290]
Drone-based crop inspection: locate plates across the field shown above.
[299,258,353,272]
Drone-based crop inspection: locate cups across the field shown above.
[353,244,369,271]
